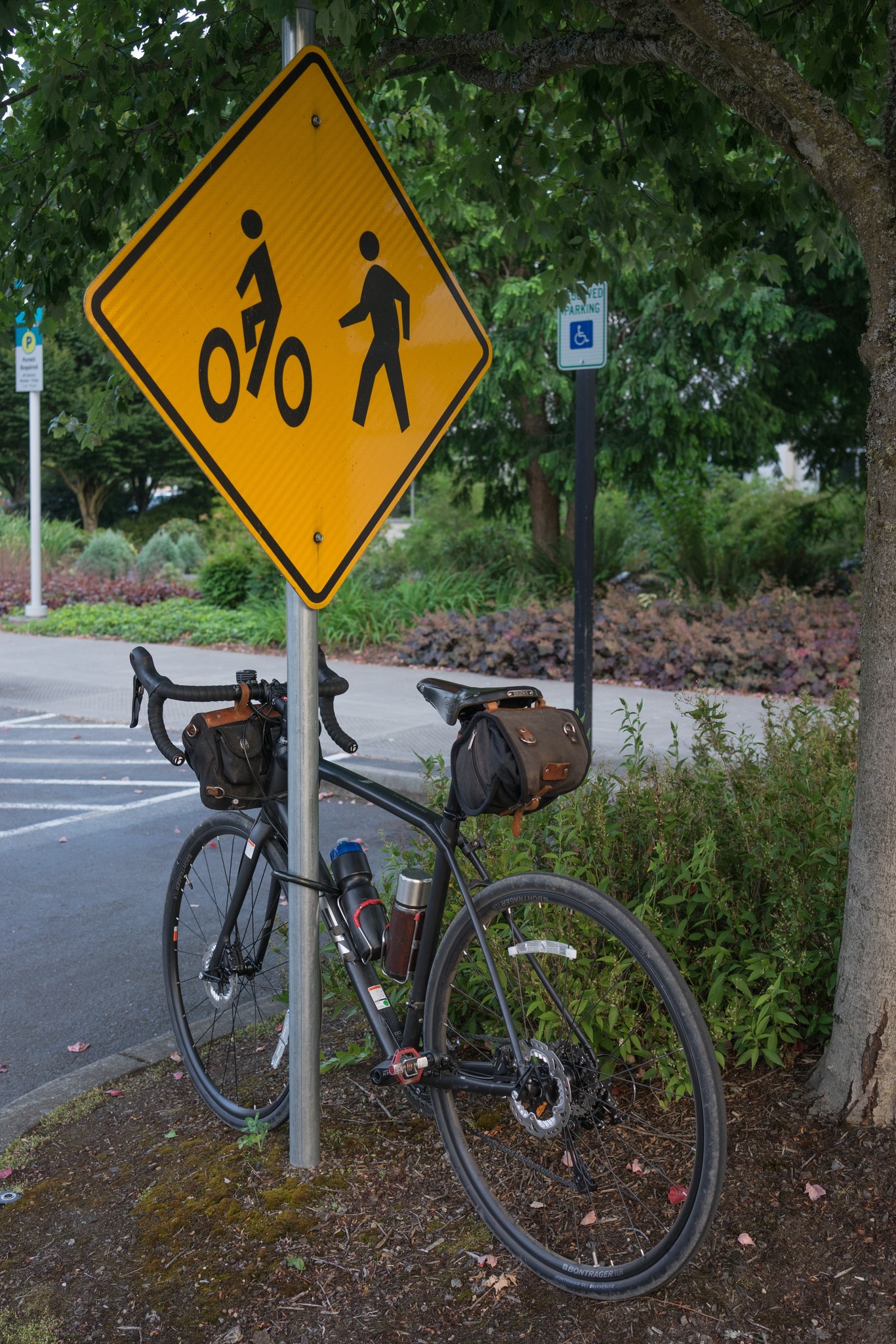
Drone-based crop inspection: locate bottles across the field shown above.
[330,838,389,960]
[383,868,432,980]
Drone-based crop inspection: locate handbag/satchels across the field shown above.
[182,684,291,810]
[451,706,589,816]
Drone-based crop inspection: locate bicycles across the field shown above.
[126,644,727,1303]
